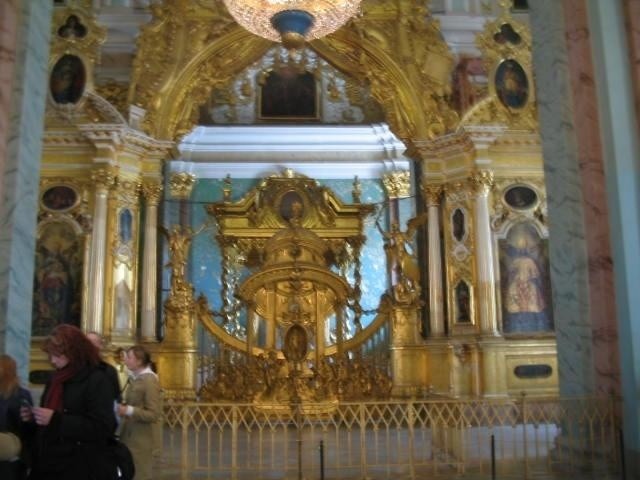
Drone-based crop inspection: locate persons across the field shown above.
[0,351,34,479]
[20,323,120,479]
[118,346,162,480]
[84,329,122,433]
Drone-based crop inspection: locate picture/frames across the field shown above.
[492,213,561,340]
[29,215,90,342]
[452,277,477,326]
[254,64,324,124]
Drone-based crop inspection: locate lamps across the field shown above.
[223,0,362,59]
